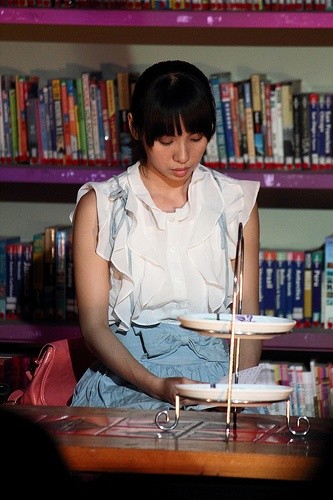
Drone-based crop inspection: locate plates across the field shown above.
[176,383,294,402]
[178,313,296,333]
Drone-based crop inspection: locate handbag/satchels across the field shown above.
[7,337,84,407]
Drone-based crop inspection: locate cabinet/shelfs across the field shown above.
[0,0,333,353]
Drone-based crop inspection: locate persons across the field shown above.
[68,59,264,415]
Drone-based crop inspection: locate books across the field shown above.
[1,72,333,174]
[254,360,333,418]
[1,224,332,337]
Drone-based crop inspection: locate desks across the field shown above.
[0,404,333,482]
